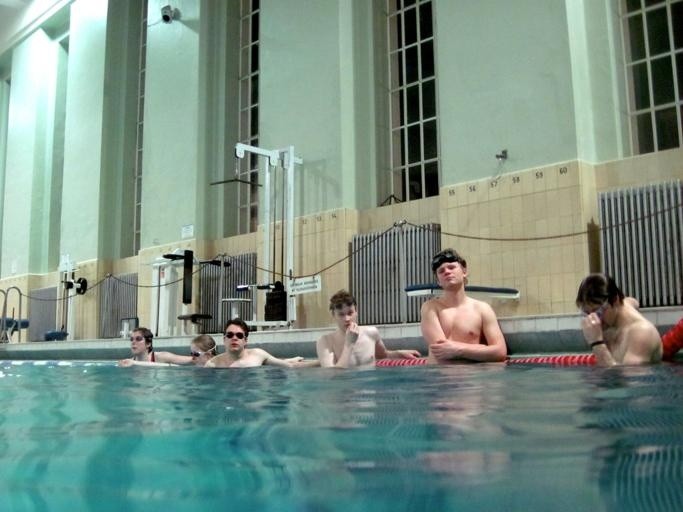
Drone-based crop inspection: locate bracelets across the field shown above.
[589,339,605,347]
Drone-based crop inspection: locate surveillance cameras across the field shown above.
[160,6,175,23]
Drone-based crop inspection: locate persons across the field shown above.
[202,318,304,367]
[574,272,664,369]
[125,327,192,365]
[115,332,215,368]
[419,247,506,366]
[313,287,421,371]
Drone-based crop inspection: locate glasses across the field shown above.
[332,299,354,312]
[190,352,206,359]
[581,299,609,318]
[224,331,246,339]
[130,336,145,343]
[432,250,458,274]
